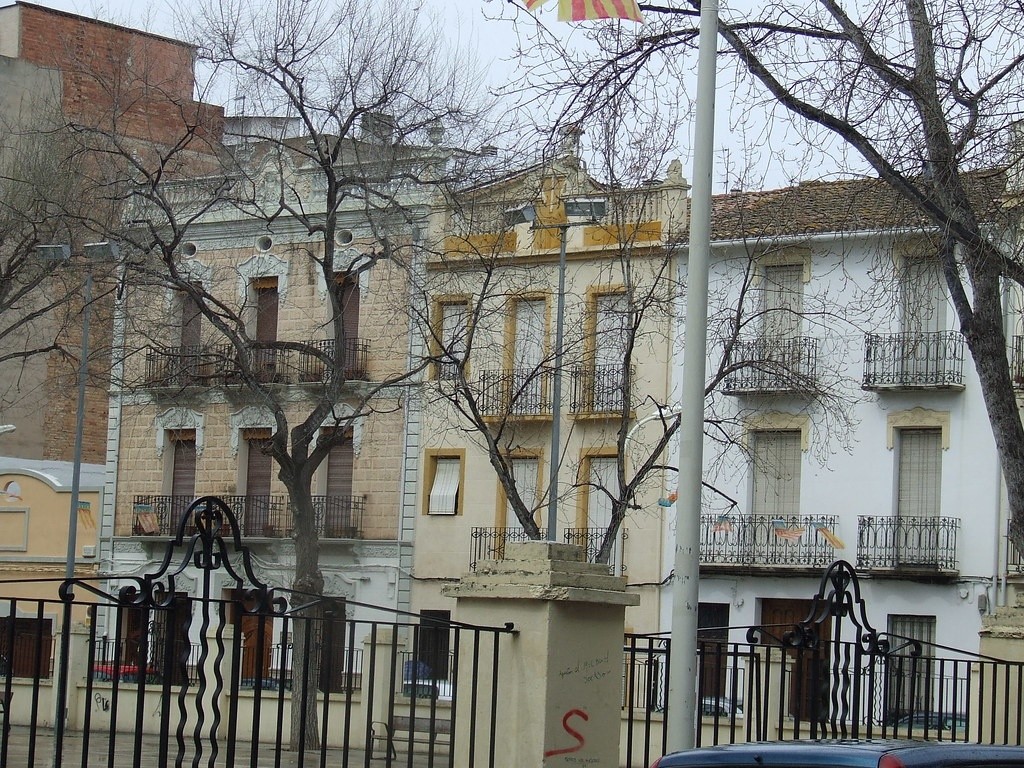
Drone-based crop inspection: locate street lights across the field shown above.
[500,198,608,541]
[37,242,120,593]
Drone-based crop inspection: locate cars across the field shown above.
[893,712,965,731]
[92,666,154,684]
[653,697,742,719]
[649,738,1024,768]
[240,678,293,692]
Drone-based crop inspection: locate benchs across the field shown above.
[371,715,452,760]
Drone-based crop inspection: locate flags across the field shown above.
[772,520,806,543]
[658,489,678,507]
[710,516,733,532]
[813,521,846,550]
